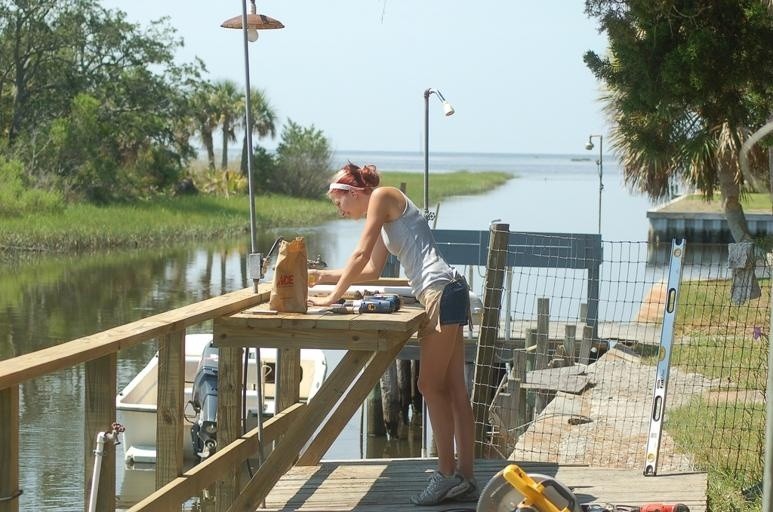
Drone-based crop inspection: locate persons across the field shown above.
[307,161,479,505]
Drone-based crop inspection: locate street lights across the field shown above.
[216,0,285,293]
[583,132,604,235]
[418,84,454,222]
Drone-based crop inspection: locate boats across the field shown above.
[109,332,331,467]
[110,463,197,512]
[568,157,591,161]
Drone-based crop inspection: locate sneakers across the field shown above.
[410,471,479,506]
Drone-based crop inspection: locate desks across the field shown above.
[212,293,428,512]
